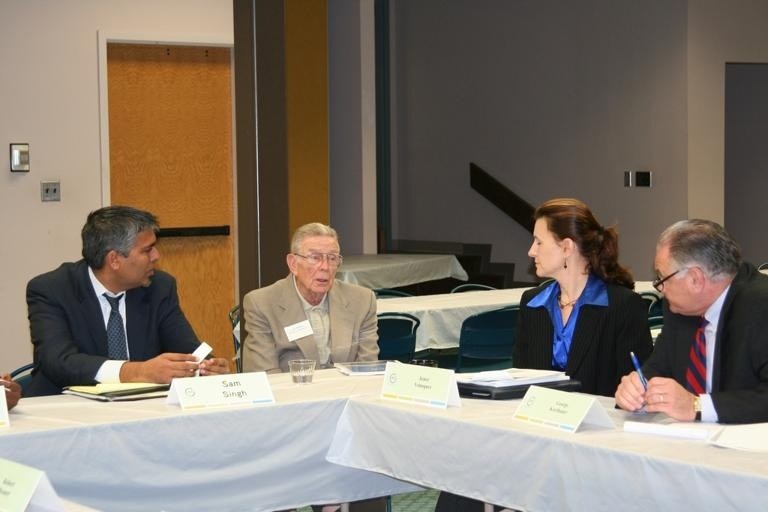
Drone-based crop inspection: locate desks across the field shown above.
[2,252,768,512]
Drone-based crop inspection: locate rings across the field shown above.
[660,394,664,402]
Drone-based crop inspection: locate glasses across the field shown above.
[293,252,341,265]
[653,266,691,292]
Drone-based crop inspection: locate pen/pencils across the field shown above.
[629,352,647,387]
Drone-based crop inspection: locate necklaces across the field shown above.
[558,297,576,309]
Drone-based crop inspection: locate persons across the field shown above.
[22,205,231,399]
[614,218,768,425]
[242,222,380,373]
[0,372,22,412]
[434,196,654,512]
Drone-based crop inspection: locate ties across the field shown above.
[103,294,128,360]
[685,317,709,395]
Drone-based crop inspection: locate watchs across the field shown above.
[693,395,704,421]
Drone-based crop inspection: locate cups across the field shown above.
[289,359,316,389]
